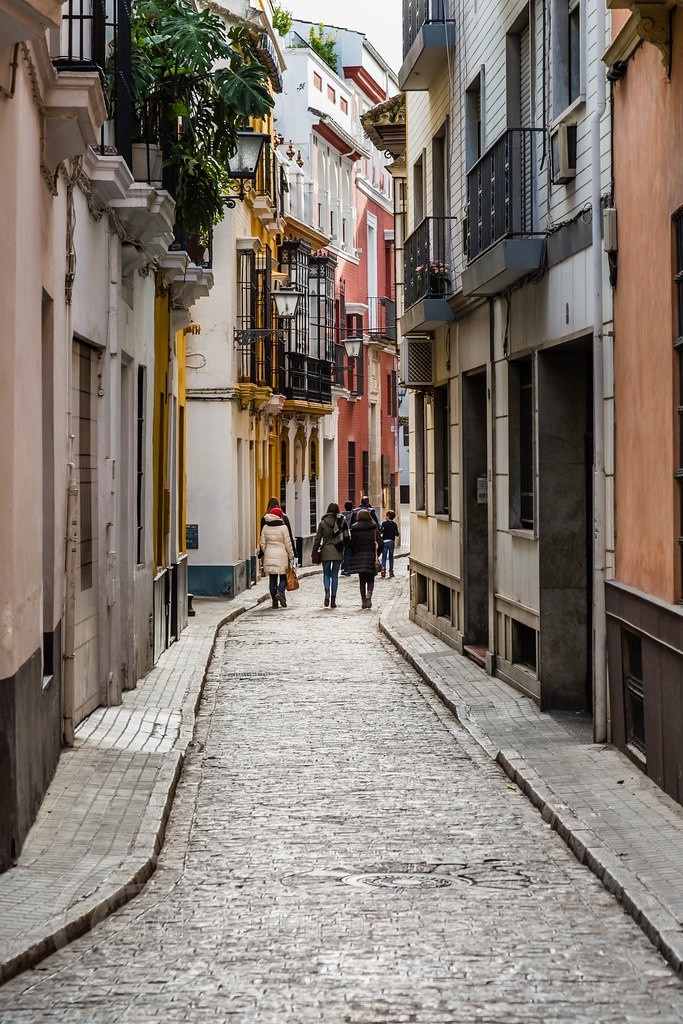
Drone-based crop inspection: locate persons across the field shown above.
[341,496,400,577]
[312,503,350,608]
[257,497,299,608]
[344,510,385,608]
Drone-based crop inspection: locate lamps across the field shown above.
[329,330,365,376]
[233,283,304,345]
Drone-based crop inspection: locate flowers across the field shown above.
[413,258,451,292]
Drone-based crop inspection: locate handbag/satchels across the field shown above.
[286,559,299,591]
[314,551,322,565]
[375,560,382,574]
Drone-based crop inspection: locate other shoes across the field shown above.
[275,593,286,607]
[272,601,278,609]
[390,574,395,577]
[381,573,386,576]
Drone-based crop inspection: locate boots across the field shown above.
[324,589,329,607]
[331,596,337,609]
[362,591,372,608]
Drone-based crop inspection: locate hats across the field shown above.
[270,508,283,517]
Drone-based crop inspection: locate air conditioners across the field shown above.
[399,340,435,386]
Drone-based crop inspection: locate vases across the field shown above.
[422,276,444,298]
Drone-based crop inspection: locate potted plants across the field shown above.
[107,0,275,263]
